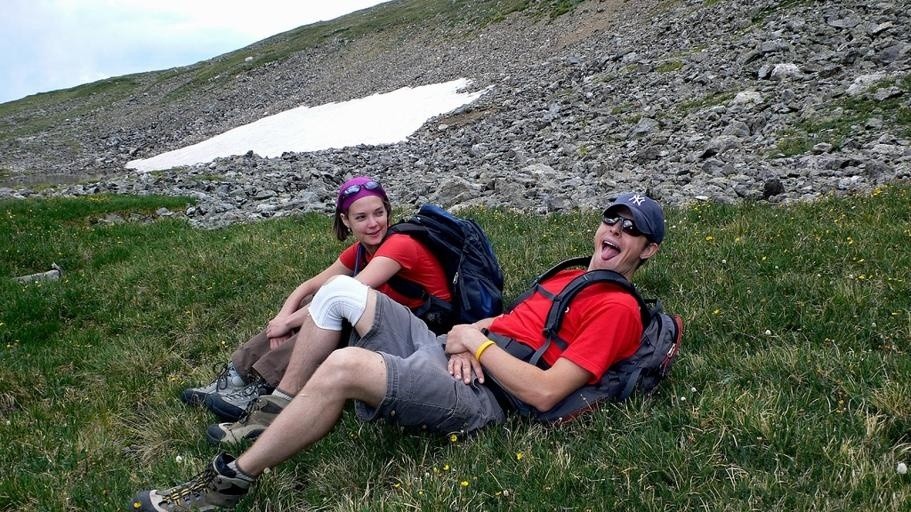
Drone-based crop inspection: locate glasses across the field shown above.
[338,181,384,208]
[602,209,640,237]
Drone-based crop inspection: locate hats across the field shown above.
[334,178,387,229]
[600,192,665,244]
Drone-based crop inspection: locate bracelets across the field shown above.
[475,339,497,361]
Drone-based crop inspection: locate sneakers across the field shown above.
[206,374,274,420]
[206,394,291,448]
[128,452,259,511]
[178,360,245,409]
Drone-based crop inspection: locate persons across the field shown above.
[129,191,667,512]
[179,177,459,422]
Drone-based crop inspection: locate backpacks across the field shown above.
[386,202,505,322]
[510,256,683,424]
